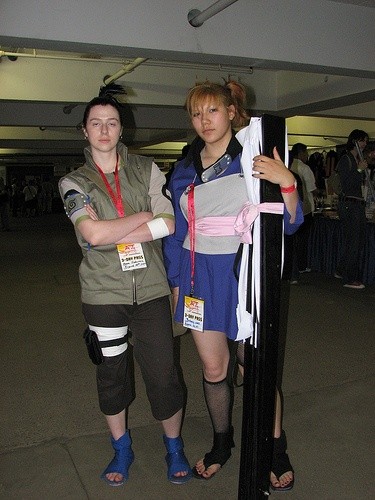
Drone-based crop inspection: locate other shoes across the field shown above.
[269,430,295,491]
[163,434,191,484]
[299,268,311,273]
[334,271,344,279]
[287,278,298,284]
[100,430,134,487]
[191,427,233,480]
[344,279,365,288]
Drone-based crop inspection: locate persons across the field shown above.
[158,82,306,490]
[0,176,61,231]
[57,96,193,484]
[286,129,375,289]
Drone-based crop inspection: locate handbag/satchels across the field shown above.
[327,154,352,195]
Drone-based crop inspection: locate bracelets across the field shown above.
[280,179,298,193]
[357,168,363,173]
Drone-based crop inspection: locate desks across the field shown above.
[311,212,375,285]
